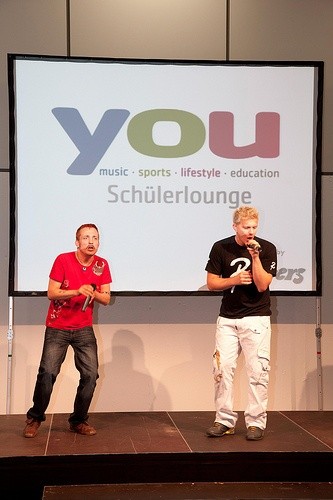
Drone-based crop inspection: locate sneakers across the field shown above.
[23,416,41,438]
[246,426,265,440]
[71,421,95,435]
[208,421,235,437]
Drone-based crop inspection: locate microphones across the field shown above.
[81,283,96,312]
[247,244,262,252]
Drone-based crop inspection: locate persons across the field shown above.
[23,223,113,438]
[204,206,278,441]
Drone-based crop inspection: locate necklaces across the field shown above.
[77,251,94,271]
